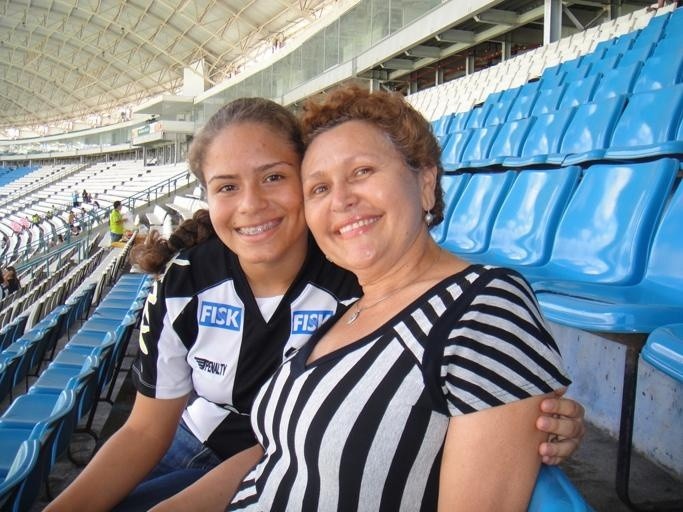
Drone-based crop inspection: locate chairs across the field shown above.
[1,163,195,256]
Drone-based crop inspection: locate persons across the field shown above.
[0,236,23,305]
[110,201,128,248]
[145,82,571,511]
[41,96,585,511]
[29,187,100,249]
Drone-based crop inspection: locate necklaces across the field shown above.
[346,245,444,326]
